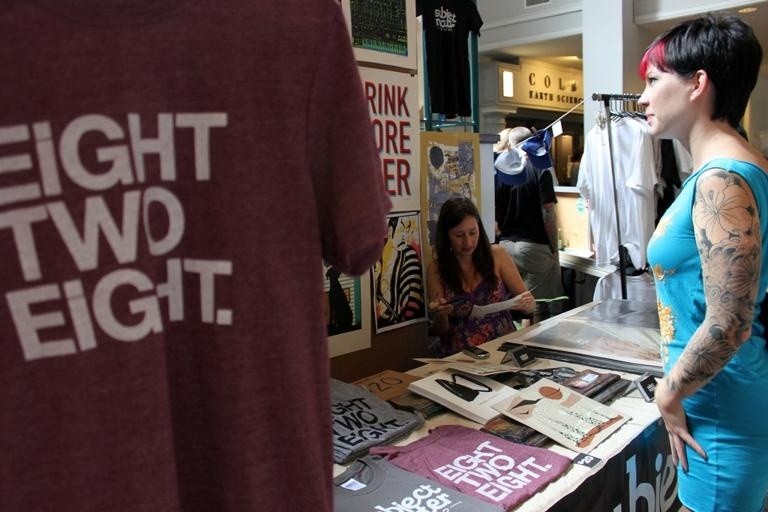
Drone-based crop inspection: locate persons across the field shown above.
[494,127,566,324]
[492,128,511,162]
[570,146,584,187]
[425,196,537,356]
[635,12,768,511]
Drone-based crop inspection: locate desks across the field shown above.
[332,300,692,511]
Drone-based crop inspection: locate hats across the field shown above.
[522,129,553,168]
[494,148,527,185]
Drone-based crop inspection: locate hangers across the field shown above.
[590,91,650,134]
[599,254,656,290]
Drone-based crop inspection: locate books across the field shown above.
[490,377,634,454]
